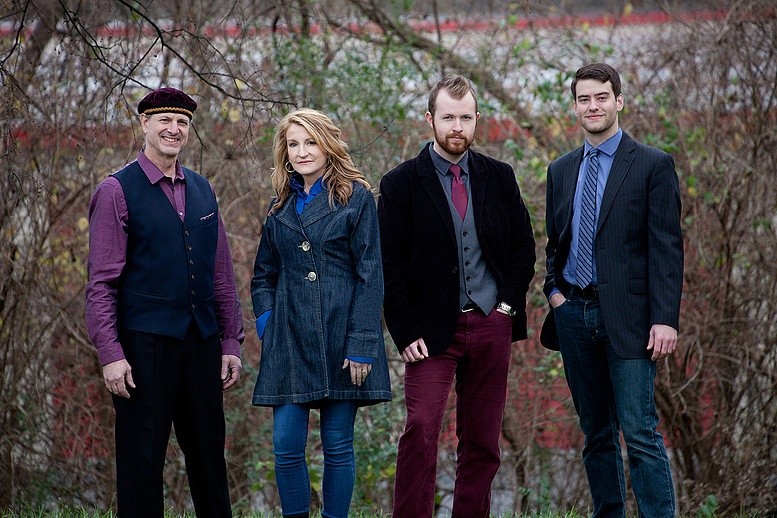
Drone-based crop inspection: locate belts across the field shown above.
[560,276,600,300]
[460,303,479,312]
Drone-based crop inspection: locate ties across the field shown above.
[448,163,469,222]
[574,147,600,291]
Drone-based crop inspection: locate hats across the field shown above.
[138,87,198,121]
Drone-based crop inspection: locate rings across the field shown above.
[362,370,366,373]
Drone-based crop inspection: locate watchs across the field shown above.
[497,303,516,317]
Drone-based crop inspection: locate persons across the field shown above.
[377,74,536,518]
[250,109,393,518]
[543,64,684,518]
[85,88,246,518]
